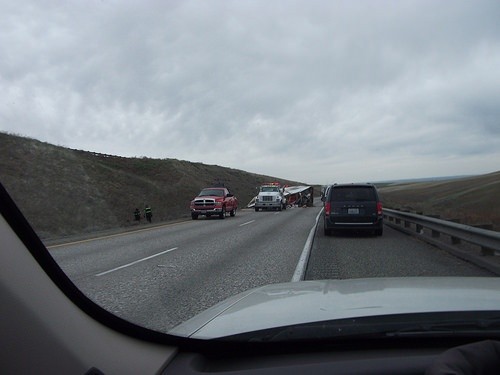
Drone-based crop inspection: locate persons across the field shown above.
[134,208,142,221]
[144,205,153,223]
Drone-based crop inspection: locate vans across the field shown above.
[321,182,384,235]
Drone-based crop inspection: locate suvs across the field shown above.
[254,183,287,211]
[190,187,239,219]
